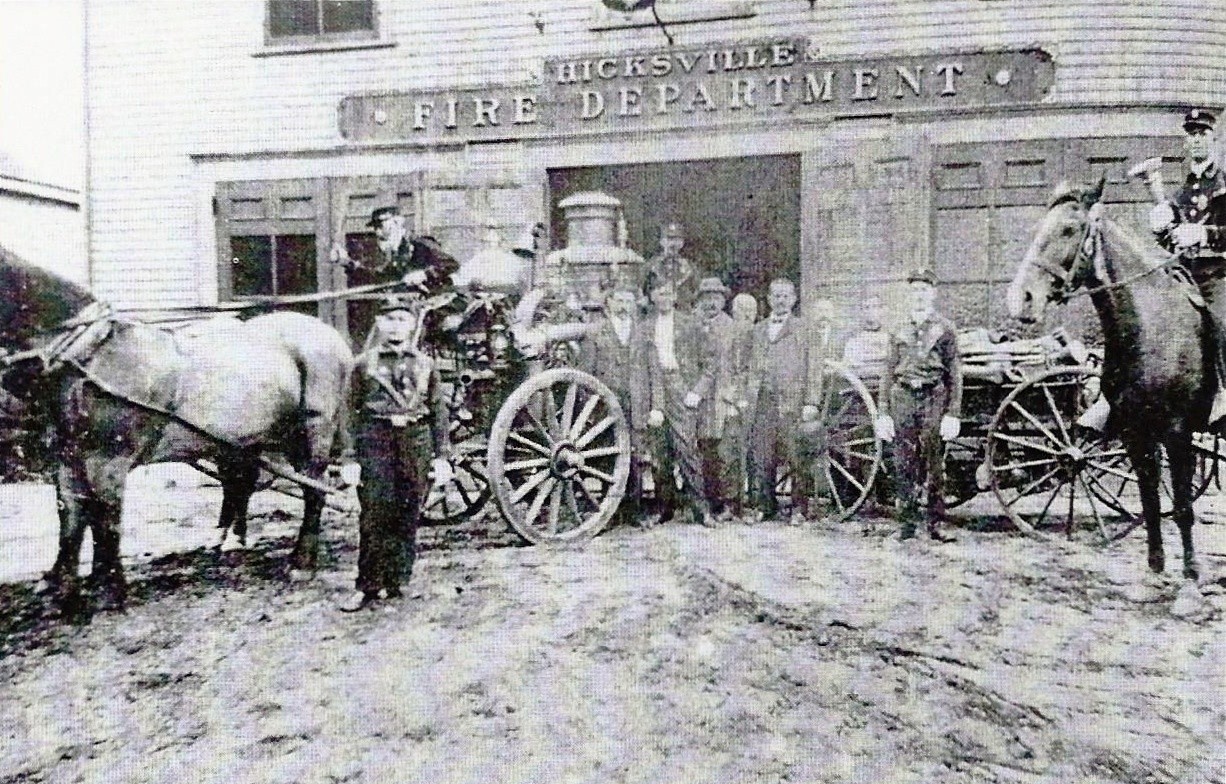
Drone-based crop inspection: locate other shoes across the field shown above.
[931,529,956,542]
[615,501,764,531]
[343,590,370,612]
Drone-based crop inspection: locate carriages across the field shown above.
[1,242,633,654]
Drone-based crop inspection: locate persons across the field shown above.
[639,222,704,318]
[1074,107,1226,433]
[874,268,963,544]
[334,205,448,347]
[579,274,893,526]
[339,296,452,613]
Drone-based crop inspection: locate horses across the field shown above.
[1001,162,1226,620]
[0,238,357,630]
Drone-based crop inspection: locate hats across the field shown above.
[697,277,731,299]
[379,295,417,316]
[907,269,938,286]
[1182,111,1216,130]
[662,223,688,237]
[365,205,403,228]
